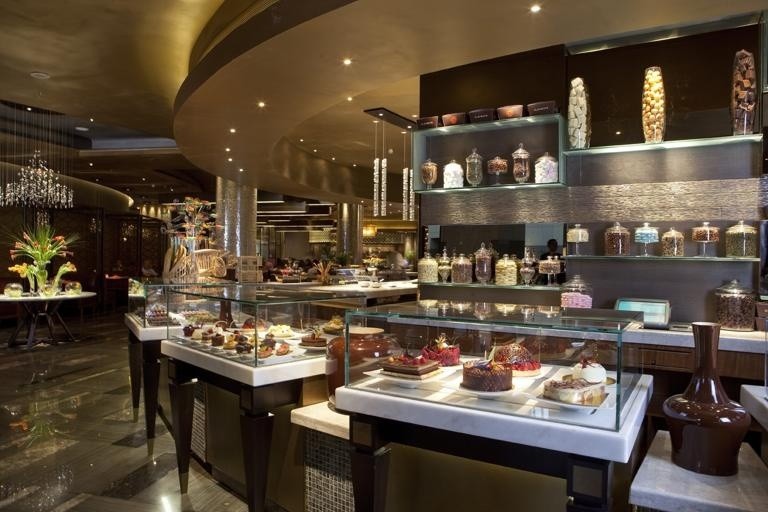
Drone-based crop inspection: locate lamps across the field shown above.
[372,115,416,222]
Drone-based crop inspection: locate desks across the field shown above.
[0,290,97,351]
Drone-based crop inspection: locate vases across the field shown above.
[662,321,752,476]
[36,270,49,294]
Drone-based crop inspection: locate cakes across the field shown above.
[378,336,608,407]
[182,316,345,358]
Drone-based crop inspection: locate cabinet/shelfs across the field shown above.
[120,282,655,510]
[413,110,767,436]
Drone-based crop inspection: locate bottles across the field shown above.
[712,274,757,331]
[418,241,563,288]
[568,220,758,259]
[420,141,558,191]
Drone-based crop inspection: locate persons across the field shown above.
[392,243,404,270]
[141,257,159,277]
[539,238,565,286]
[262,261,278,282]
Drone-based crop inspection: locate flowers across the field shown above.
[7,212,78,276]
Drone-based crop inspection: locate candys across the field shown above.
[568,77,588,149]
[729,58,757,130]
[642,70,665,141]
